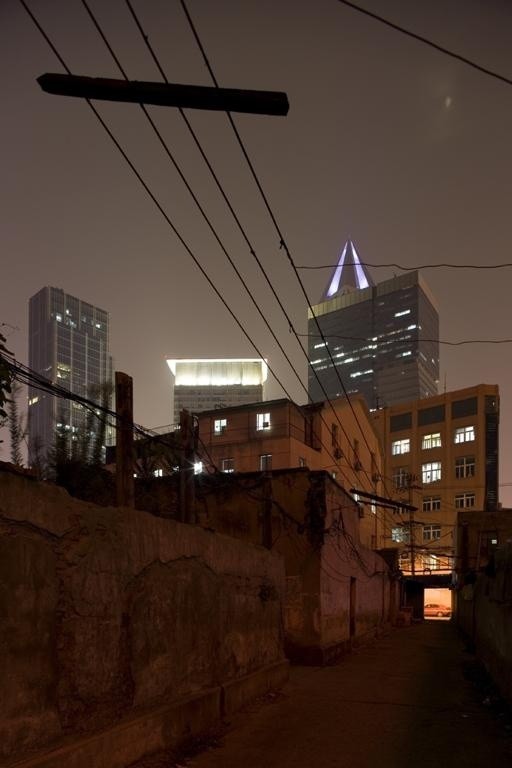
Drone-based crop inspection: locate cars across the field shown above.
[424,603,452,617]
